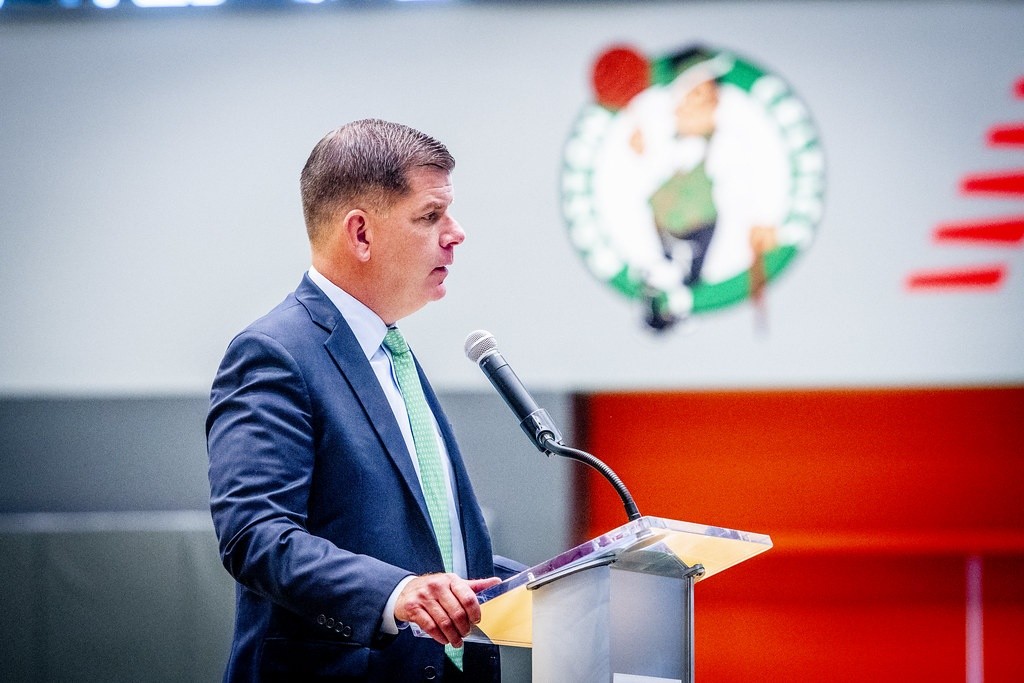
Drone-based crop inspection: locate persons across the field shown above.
[205,118,501,683]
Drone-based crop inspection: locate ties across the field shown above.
[382,327,464,674]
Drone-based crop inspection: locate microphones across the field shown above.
[463,330,566,458]
